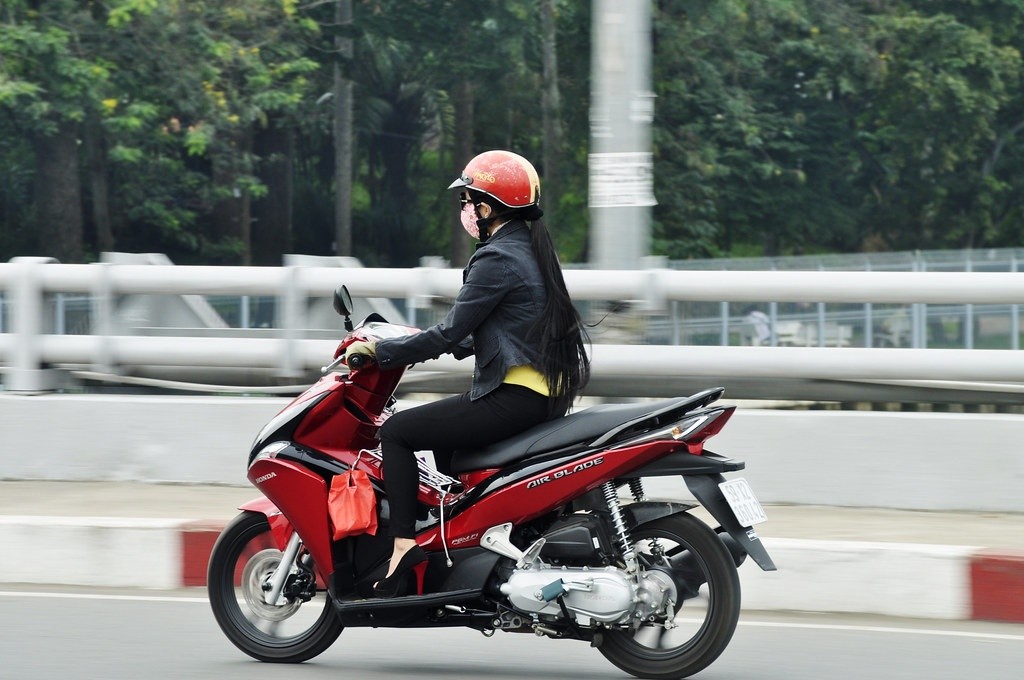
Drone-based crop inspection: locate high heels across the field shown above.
[371,545,428,599]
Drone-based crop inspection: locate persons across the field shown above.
[346,149,591,598]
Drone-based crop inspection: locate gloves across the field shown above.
[343,341,377,365]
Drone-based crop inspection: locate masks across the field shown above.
[459,202,492,239]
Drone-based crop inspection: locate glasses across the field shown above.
[458,192,480,209]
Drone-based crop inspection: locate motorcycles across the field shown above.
[207,286,779,679]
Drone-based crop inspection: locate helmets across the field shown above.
[446,150,541,209]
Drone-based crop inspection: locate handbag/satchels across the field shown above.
[326,468,378,540]
